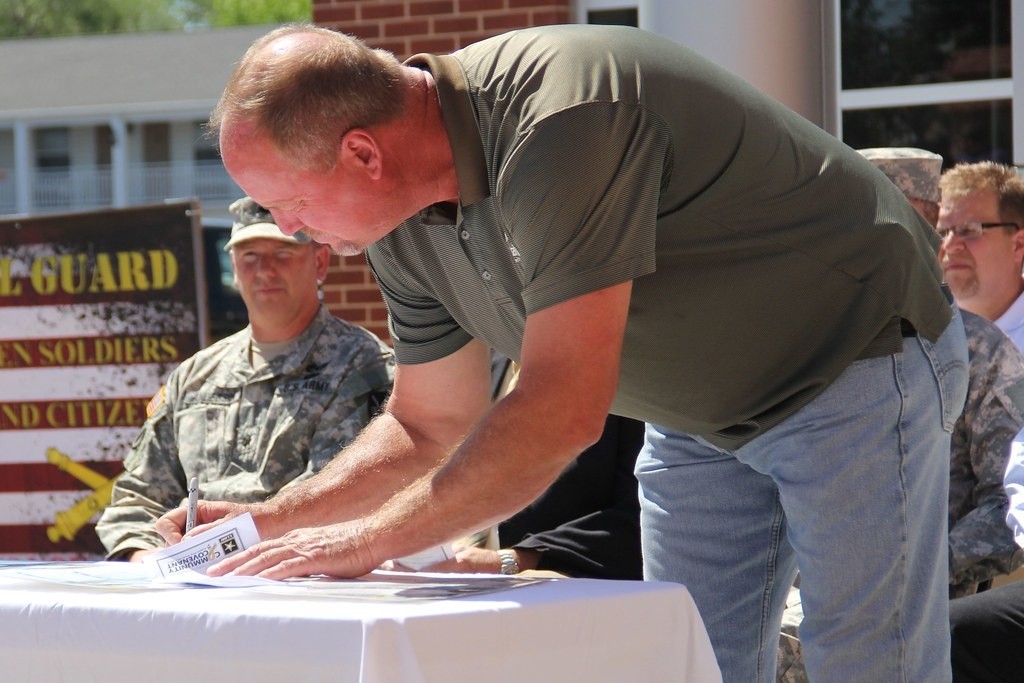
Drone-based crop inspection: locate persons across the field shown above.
[94,195,396,563]
[155,23,970,683]
[377,346,645,581]
[777,147,1024,683]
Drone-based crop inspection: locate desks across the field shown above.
[0,563,724,683]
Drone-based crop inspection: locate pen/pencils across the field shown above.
[185,477,199,533]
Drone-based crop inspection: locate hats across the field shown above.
[853,147,942,205]
[223,197,312,253]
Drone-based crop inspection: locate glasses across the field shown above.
[939,220,1023,242]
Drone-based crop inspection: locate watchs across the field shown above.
[497,550,520,575]
